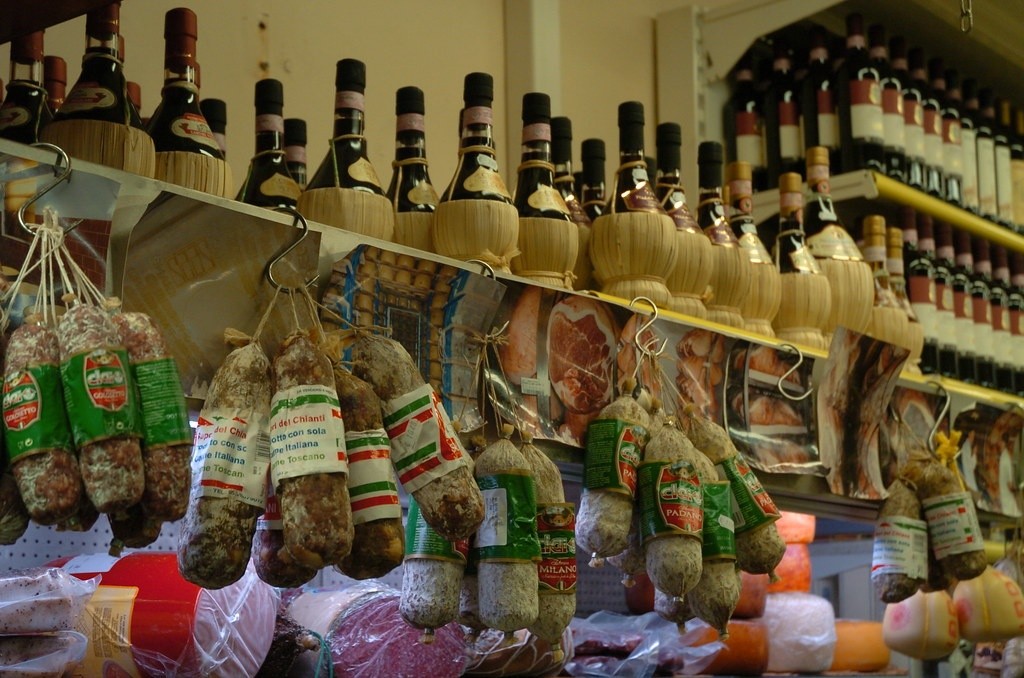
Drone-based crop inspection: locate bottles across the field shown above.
[0,0,1024,392]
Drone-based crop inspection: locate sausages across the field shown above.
[0,303,986,642]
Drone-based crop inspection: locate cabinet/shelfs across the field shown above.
[1,136,946,678]
[656,0,1024,562]
